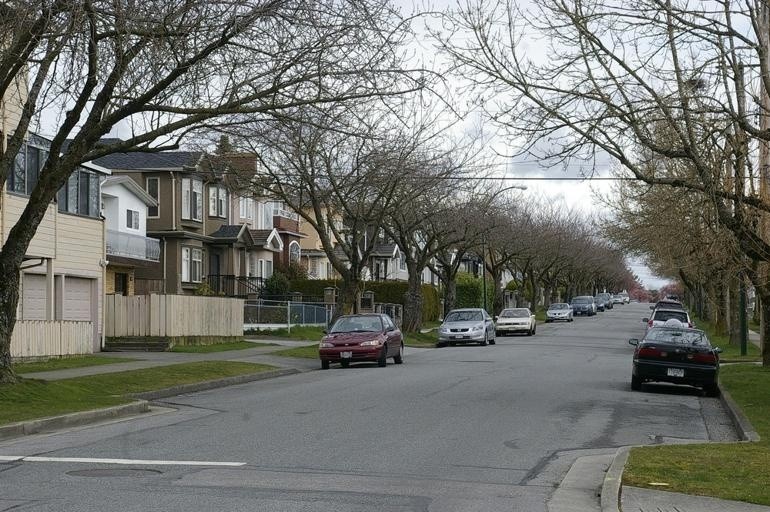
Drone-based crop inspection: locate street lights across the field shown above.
[480,183,530,312]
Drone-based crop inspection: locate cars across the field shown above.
[495,307,537,337]
[629,325,723,398]
[545,290,640,323]
[436,308,497,350]
[317,313,405,369]
[642,293,695,335]
